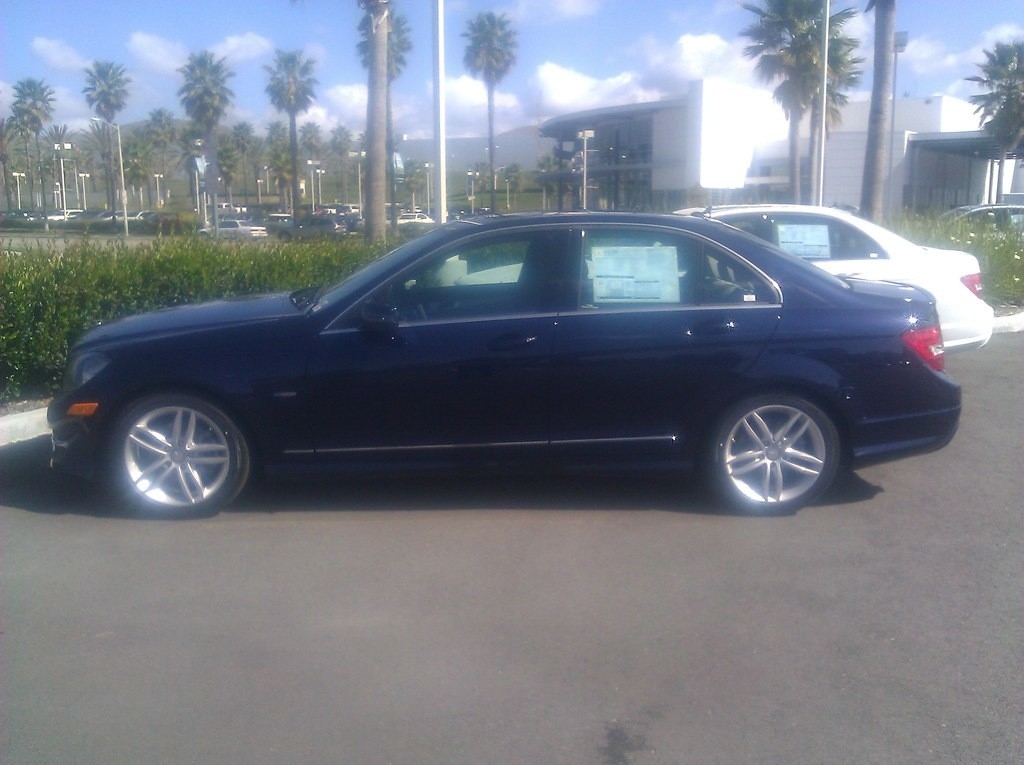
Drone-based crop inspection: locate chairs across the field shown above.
[514,244,576,315]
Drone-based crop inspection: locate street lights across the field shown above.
[92,117,130,238]
[193,138,203,214]
[79,173,90,208]
[467,169,479,215]
[317,169,326,205]
[577,129,595,210]
[52,191,59,209]
[425,163,431,217]
[54,143,72,220]
[12,172,26,209]
[256,176,264,205]
[347,151,366,220]
[306,160,320,212]
[154,174,164,208]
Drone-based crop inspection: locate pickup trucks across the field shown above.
[206,202,248,214]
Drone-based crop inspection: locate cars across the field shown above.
[409,203,994,357]
[322,203,435,225]
[0,209,154,222]
[278,215,347,242]
[43,214,962,519]
[198,220,268,240]
[934,202,1024,238]
[266,213,292,224]
